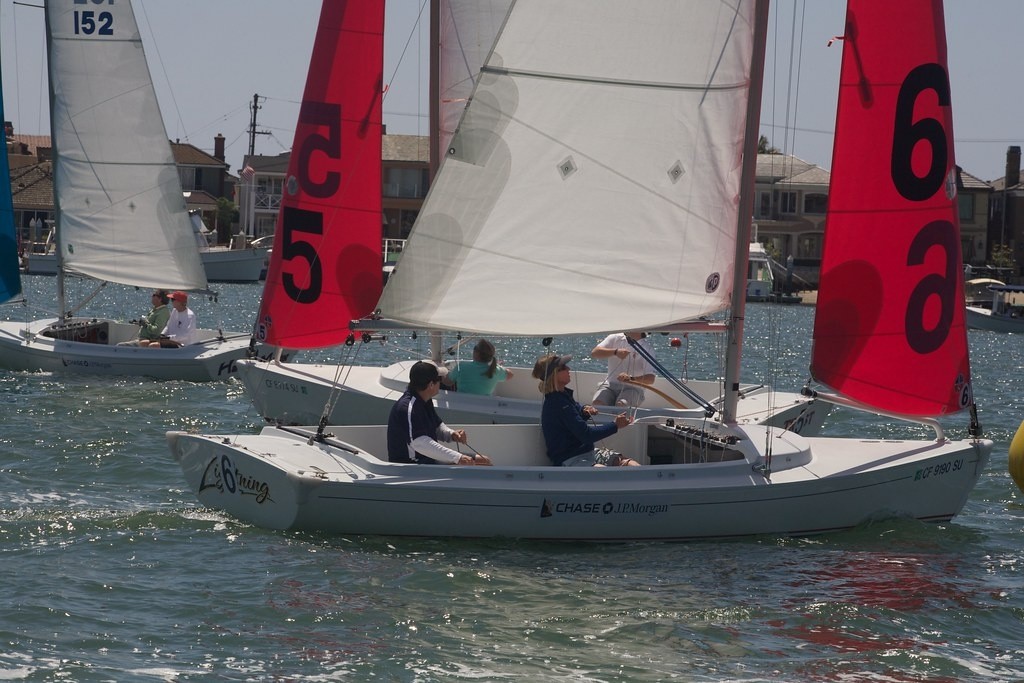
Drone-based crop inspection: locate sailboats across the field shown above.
[164,0,994,535]
[235,0,835,443]
[0,0,297,383]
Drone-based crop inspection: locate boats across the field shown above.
[745,240,778,299]
[22,203,272,282]
[959,277,1024,335]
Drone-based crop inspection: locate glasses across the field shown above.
[153,293,159,298]
[473,346,477,351]
[434,375,443,385]
[171,298,175,301]
[559,364,567,372]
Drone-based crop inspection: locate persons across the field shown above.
[387,360,493,466]
[115,288,170,346]
[532,354,641,467]
[139,291,195,349]
[442,339,514,397]
[592,332,657,406]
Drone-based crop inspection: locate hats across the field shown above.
[409,361,449,386]
[475,339,496,356]
[167,291,187,304]
[539,353,574,381]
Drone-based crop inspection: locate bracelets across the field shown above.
[614,349,618,355]
[630,375,633,380]
